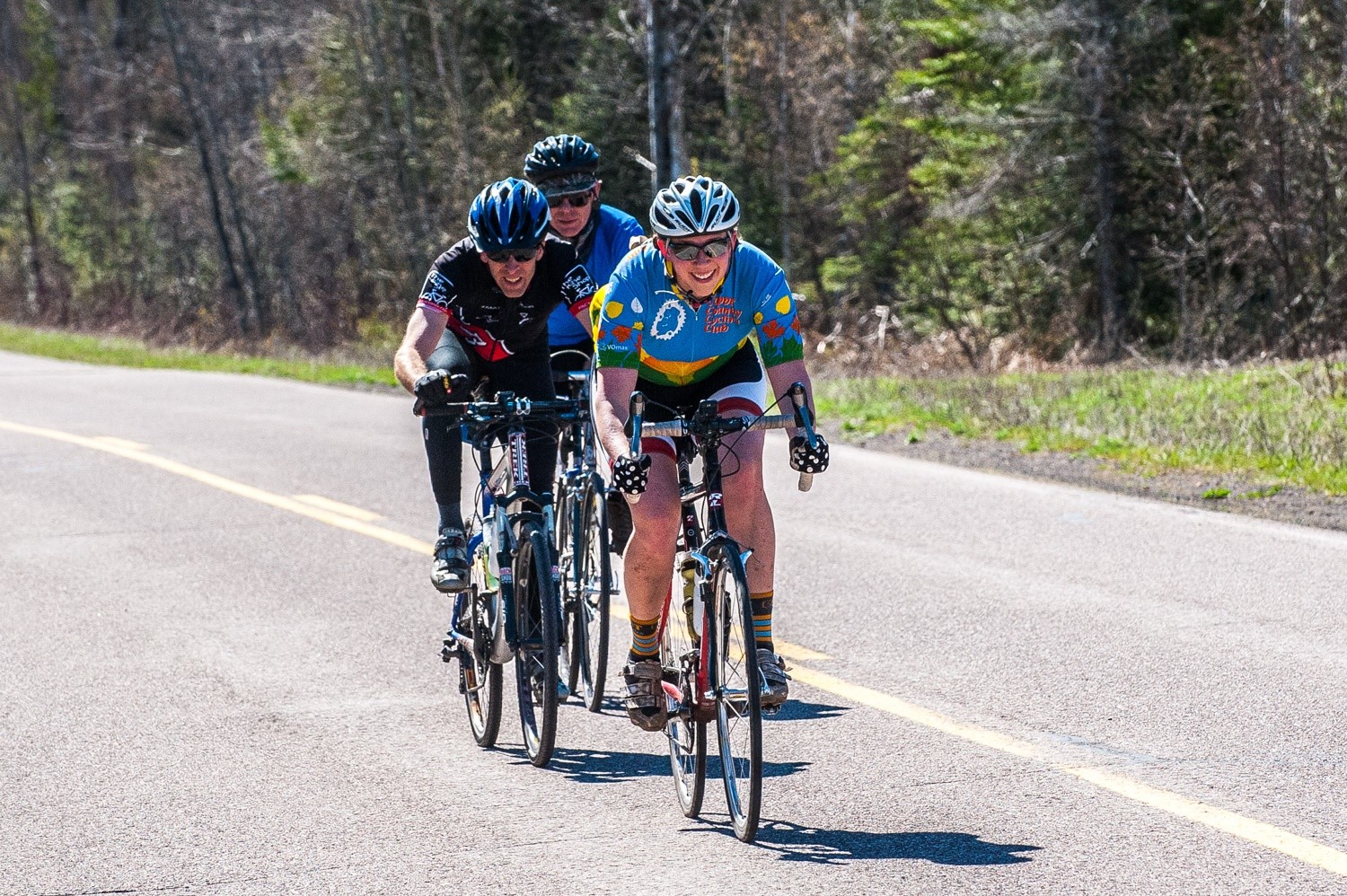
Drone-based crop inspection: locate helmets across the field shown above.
[523,134,600,184]
[467,176,552,253]
[648,175,742,238]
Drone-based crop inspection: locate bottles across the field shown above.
[681,549,702,643]
[693,575,706,638]
[481,515,499,589]
[488,506,500,581]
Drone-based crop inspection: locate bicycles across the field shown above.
[412,367,621,767]
[625,383,818,843]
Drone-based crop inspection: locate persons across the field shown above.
[590,177,828,731]
[394,176,603,705]
[525,135,649,400]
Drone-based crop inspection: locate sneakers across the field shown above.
[429,527,470,594]
[522,644,569,706]
[623,656,669,733]
[754,647,789,709]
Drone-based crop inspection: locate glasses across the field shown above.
[546,192,591,208]
[663,233,734,261]
[486,248,537,262]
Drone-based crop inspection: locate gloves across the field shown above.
[789,434,830,475]
[610,453,652,494]
[413,368,452,405]
